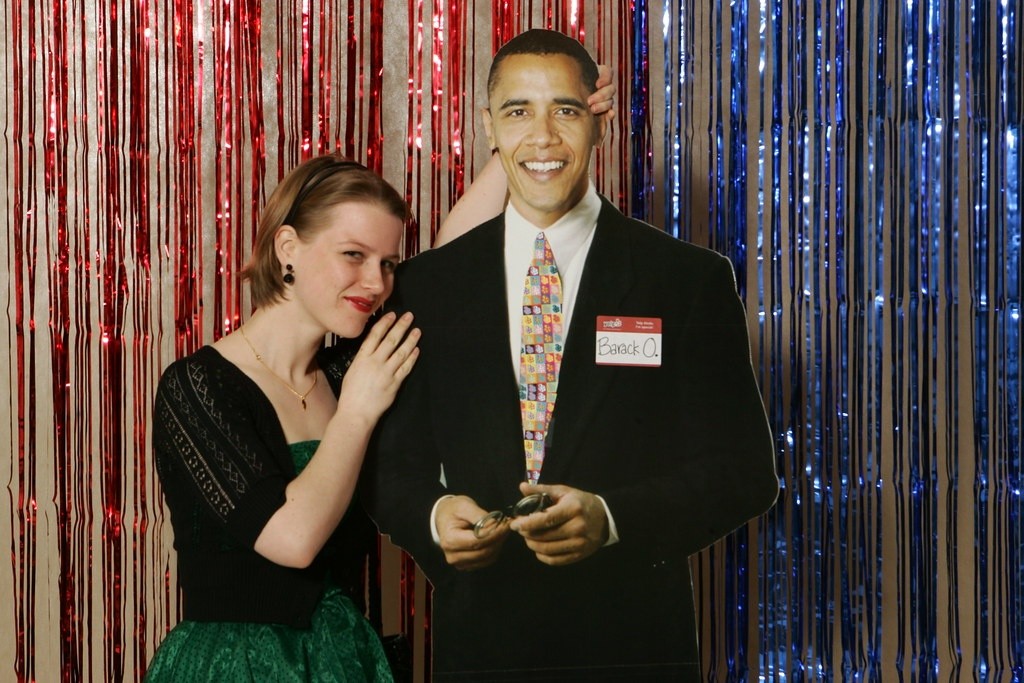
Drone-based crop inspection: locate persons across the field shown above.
[377,28,779,683]
[139,63,617,683]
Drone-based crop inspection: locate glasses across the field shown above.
[473,491,553,539]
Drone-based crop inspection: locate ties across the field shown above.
[518,232,563,486]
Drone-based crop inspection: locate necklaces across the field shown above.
[240,325,317,411]
